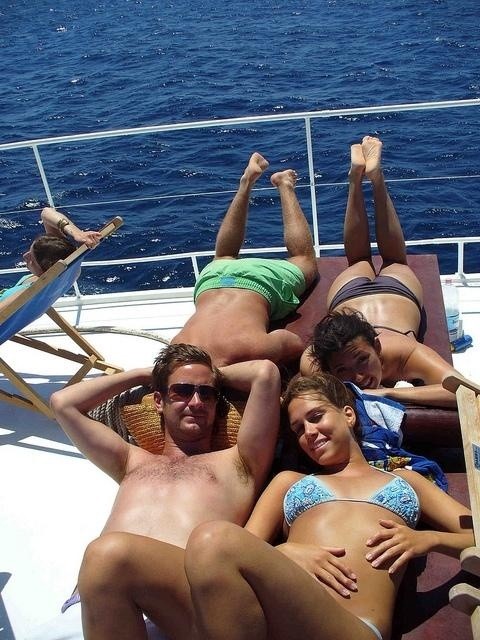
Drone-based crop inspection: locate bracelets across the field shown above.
[57,219,69,236]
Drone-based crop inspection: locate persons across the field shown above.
[42,341,283,639]
[165,150,319,371]
[297,133,467,408]
[0,206,102,302]
[76,372,476,640]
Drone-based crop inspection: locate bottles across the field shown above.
[442,278,460,341]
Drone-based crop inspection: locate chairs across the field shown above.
[268,253,480,640]
[0,216,124,426]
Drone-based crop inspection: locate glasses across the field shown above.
[162,383,218,404]
